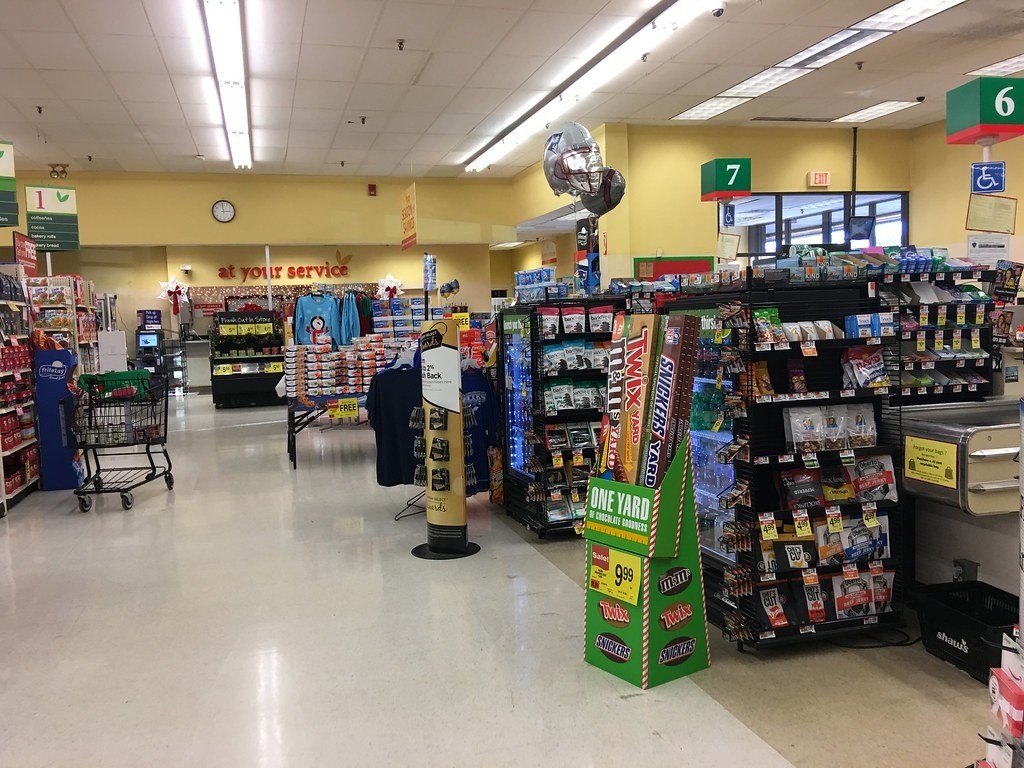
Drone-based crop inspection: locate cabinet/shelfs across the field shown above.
[0,272,118,517]
[208,353,289,409]
[495,269,1023,689]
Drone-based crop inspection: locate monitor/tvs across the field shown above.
[845,215,875,240]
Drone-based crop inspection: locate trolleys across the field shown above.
[65,347,176,511]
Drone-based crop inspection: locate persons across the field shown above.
[1013,266,1023,289]
[992,311,1005,337]
[1002,311,1014,336]
[1001,267,1015,289]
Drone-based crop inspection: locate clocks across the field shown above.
[211,199,235,223]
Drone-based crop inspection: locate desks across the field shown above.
[287,392,368,470]
